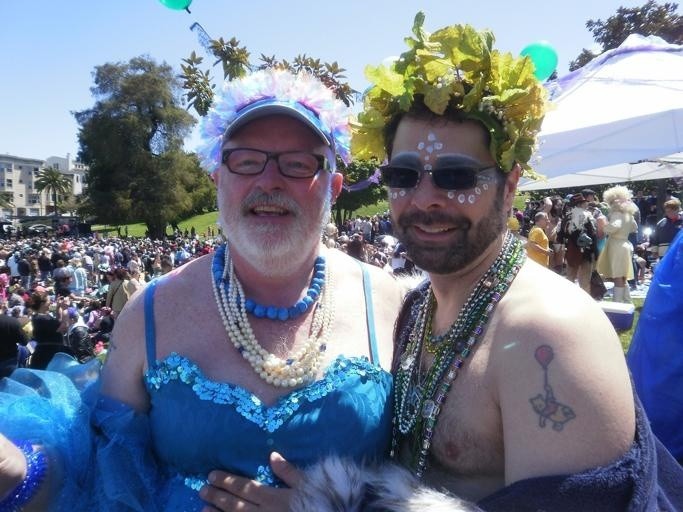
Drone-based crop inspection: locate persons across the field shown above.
[1,183,229,377]
[200,8,683,511]
[0,65,406,511]
[322,210,426,279]
[510,183,682,302]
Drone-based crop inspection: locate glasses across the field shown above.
[376,163,490,191]
[220,147,328,180]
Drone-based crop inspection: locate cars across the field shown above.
[27,223,53,234]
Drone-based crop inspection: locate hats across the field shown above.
[566,193,590,207]
[68,256,82,268]
[222,97,333,146]
[123,277,141,301]
